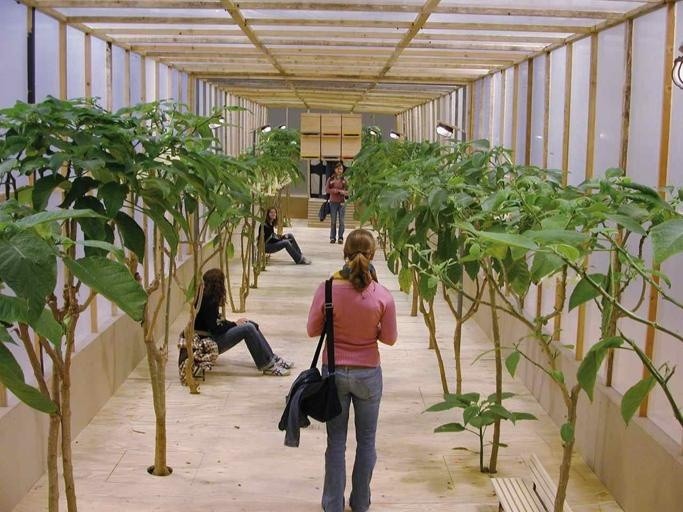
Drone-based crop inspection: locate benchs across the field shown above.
[181,322,206,381]
[254,240,270,265]
[489,451,571,512]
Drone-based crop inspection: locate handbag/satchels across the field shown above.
[300,367,342,422]
[324,202,331,215]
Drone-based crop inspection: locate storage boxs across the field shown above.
[299,112,363,160]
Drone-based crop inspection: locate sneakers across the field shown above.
[263,363,292,376]
[300,255,312,265]
[272,354,294,369]
[330,238,344,244]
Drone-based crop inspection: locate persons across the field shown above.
[306,228,399,511]
[194,266,295,376]
[258,207,312,264]
[324,162,348,245]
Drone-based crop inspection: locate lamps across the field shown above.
[278,124,286,130]
[388,130,405,140]
[436,120,465,138]
[209,116,226,129]
[369,129,378,135]
[253,124,272,156]
